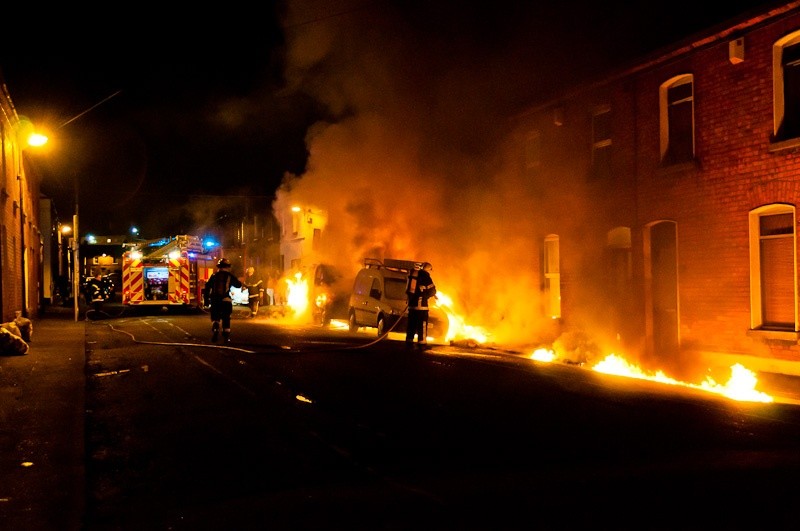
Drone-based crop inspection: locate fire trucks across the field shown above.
[119,234,250,315]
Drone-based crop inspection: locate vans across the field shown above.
[346,255,449,343]
[306,263,356,329]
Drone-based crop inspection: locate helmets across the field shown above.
[246,267,255,273]
[422,263,432,271]
[218,258,231,268]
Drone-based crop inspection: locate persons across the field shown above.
[241,266,265,318]
[404,263,436,351]
[87,272,115,320]
[203,258,242,345]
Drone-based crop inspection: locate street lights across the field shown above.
[56,222,72,278]
[17,133,49,320]
